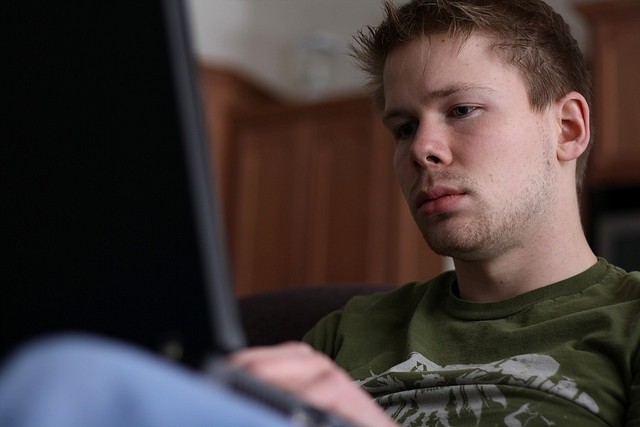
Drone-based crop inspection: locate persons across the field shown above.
[3,0,638,427]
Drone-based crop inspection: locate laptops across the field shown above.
[1,0,363,426]
